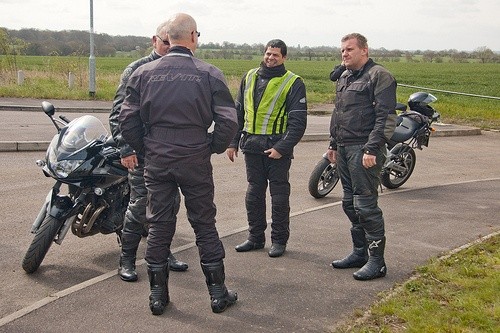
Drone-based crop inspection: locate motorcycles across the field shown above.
[309,92,441,199]
[21,101,149,274]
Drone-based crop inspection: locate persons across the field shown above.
[226,39,307,257]
[118,13,238,314]
[330,61,346,82]
[327,34,396,281]
[109,22,189,282]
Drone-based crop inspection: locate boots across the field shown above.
[332,228,369,268]
[200,261,238,312]
[167,252,188,270]
[146,261,169,315]
[353,233,387,280]
[118,231,142,280]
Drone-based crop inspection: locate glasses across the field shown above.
[192,31,200,37]
[156,35,169,45]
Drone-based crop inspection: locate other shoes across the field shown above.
[269,243,285,256]
[235,240,265,251]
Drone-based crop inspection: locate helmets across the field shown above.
[408,92,434,116]
